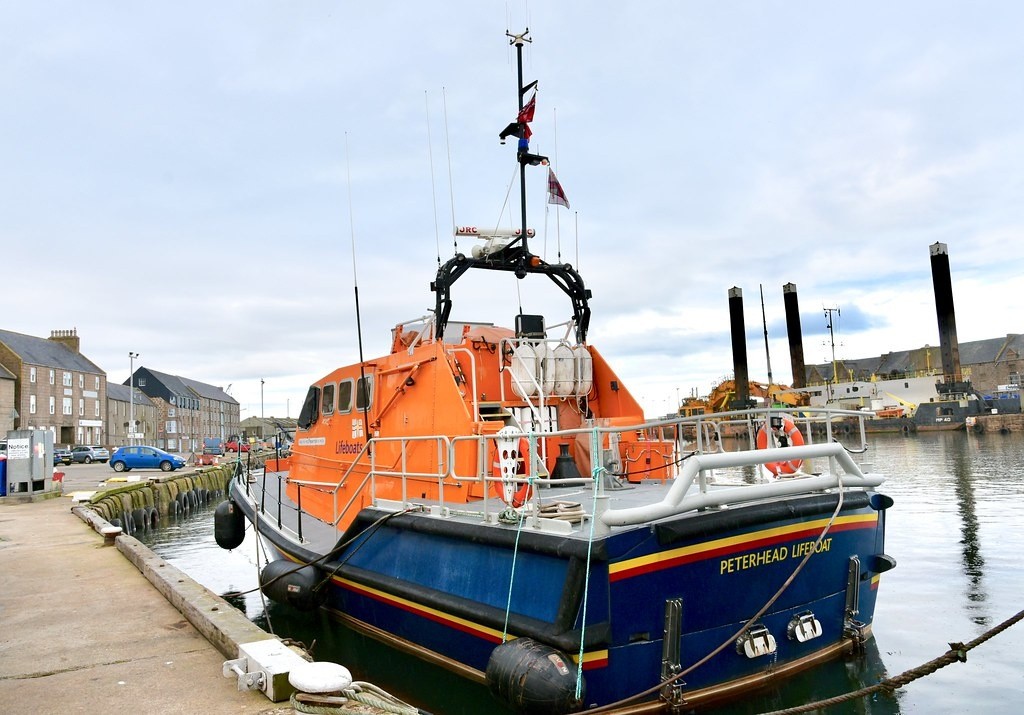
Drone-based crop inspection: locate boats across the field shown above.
[206,19,897,715]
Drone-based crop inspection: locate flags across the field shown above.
[517,90,569,208]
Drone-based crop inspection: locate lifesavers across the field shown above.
[492,436,533,509]
[756,418,805,475]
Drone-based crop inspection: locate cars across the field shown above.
[52,448,73,466]
[70,446,110,464]
[110,445,186,472]
[226,439,251,452]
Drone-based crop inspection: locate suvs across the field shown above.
[202,437,225,457]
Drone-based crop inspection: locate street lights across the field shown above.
[129,352,140,446]
[259,377,265,439]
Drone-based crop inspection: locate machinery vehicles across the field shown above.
[674,379,810,427]
